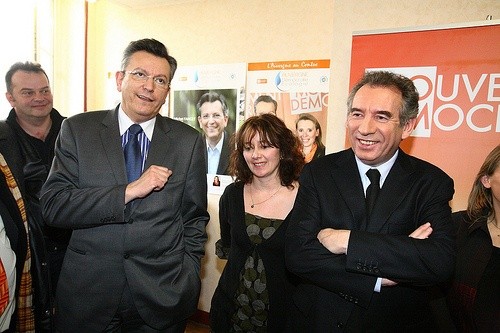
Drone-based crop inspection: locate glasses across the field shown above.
[123,71,171,87]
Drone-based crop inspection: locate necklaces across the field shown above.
[488,215,500,236]
[250,181,284,208]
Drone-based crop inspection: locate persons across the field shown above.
[196,92,235,176]
[253,95,278,116]
[213,176,220,186]
[210,71,500,333]
[295,113,325,163]
[0,60,74,333]
[38,39,210,333]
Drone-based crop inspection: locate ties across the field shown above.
[366,169,381,216]
[123,124,144,184]
